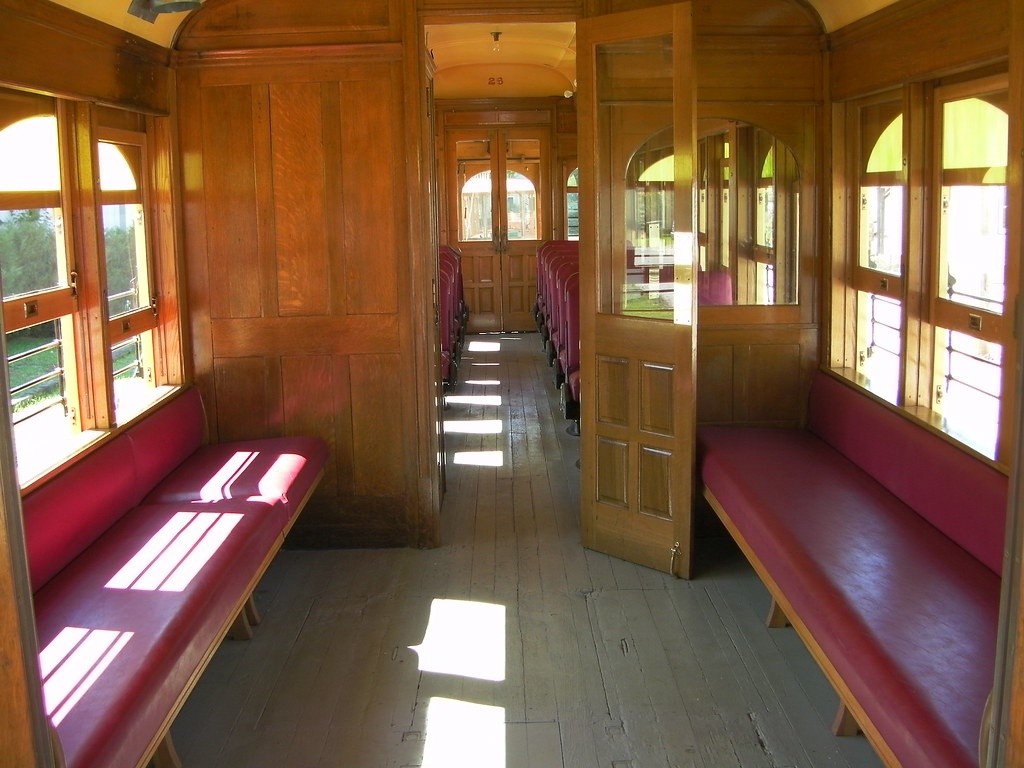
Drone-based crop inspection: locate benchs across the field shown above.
[696,364,1012,768]
[531,239,734,468]
[438,245,470,410]
[22,385,329,768]
